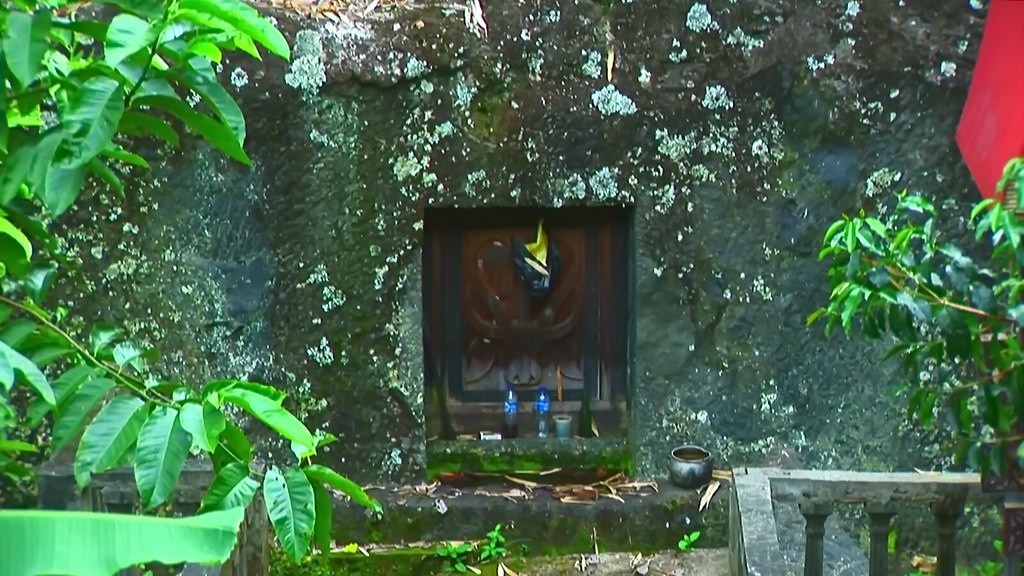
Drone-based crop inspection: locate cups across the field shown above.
[553,415,573,440]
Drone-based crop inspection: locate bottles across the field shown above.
[503,384,520,438]
[533,387,550,439]
[666,445,713,490]
[580,391,593,438]
